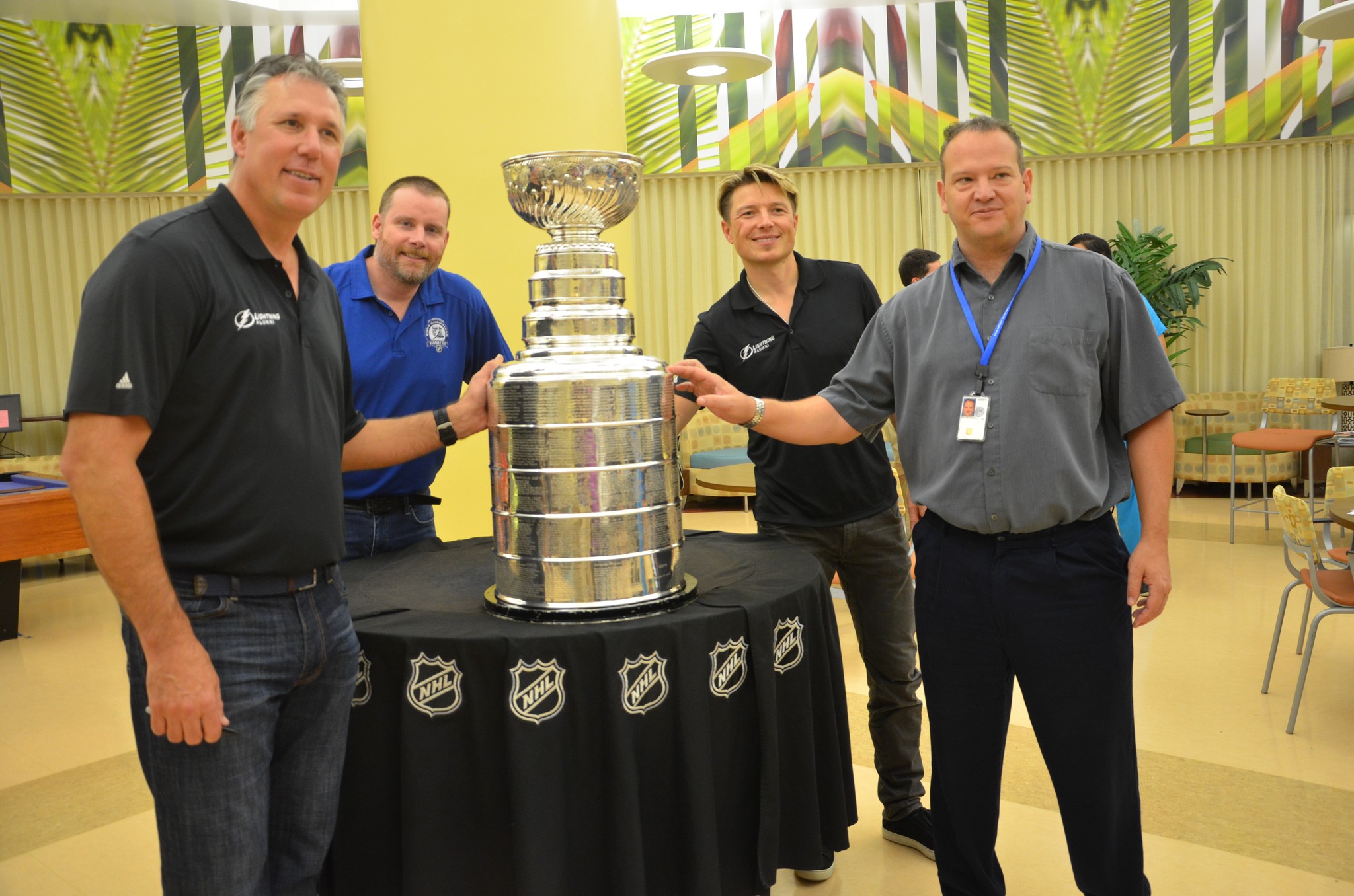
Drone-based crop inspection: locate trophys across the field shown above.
[485,149,700,626]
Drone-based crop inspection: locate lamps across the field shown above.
[316,27,364,98]
[642,11,773,86]
[1322,344,1354,434]
[1297,0,1354,41]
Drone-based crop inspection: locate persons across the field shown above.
[59,54,509,896]
[668,117,1185,896]
[321,176,516,561]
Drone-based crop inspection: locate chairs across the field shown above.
[1261,464,1354,734]
[1230,378,1340,543]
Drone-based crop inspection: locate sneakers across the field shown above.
[882,807,935,861]
[796,849,835,881]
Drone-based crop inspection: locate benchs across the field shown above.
[675,407,755,512]
[0,455,94,577]
[1172,391,1302,501]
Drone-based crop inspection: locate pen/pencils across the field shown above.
[145,706,242,737]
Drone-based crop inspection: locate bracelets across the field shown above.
[739,396,765,429]
[435,407,457,448]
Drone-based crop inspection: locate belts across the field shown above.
[175,567,325,597]
[343,489,441,514]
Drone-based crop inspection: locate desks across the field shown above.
[1185,408,1229,481]
[0,472,89,561]
[1330,496,1354,551]
[696,461,905,492]
[1321,395,1354,412]
[336,527,858,896]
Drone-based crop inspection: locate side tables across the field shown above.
[1301,444,1354,499]
